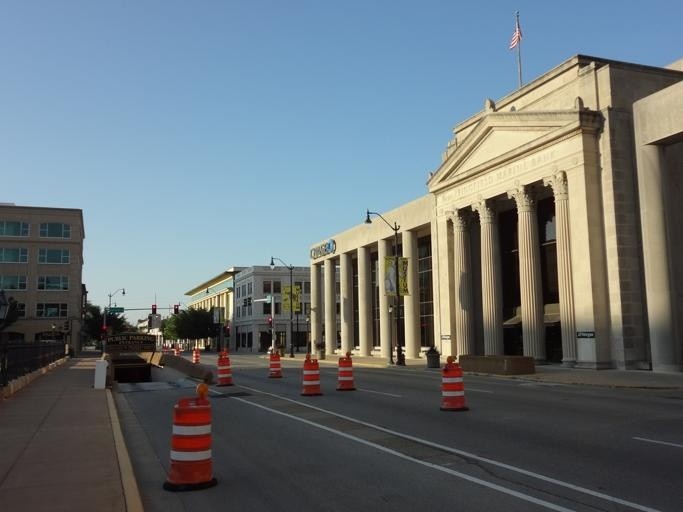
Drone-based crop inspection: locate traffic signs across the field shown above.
[109,307,124,313]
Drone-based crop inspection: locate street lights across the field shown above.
[110,301,118,307]
[363,206,408,367]
[386,303,394,365]
[269,255,296,359]
[107,288,127,307]
[51,323,56,341]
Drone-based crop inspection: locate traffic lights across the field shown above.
[151,304,156,315]
[63,321,71,331]
[243,298,246,307]
[173,304,179,315]
[100,326,108,342]
[225,325,230,339]
[246,297,252,306]
[268,317,272,329]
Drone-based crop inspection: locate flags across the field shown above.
[507,19,522,50]
[212,307,219,324]
[397,257,409,296]
[291,286,300,311]
[219,307,224,323]
[280,286,290,311]
[383,257,397,296]
